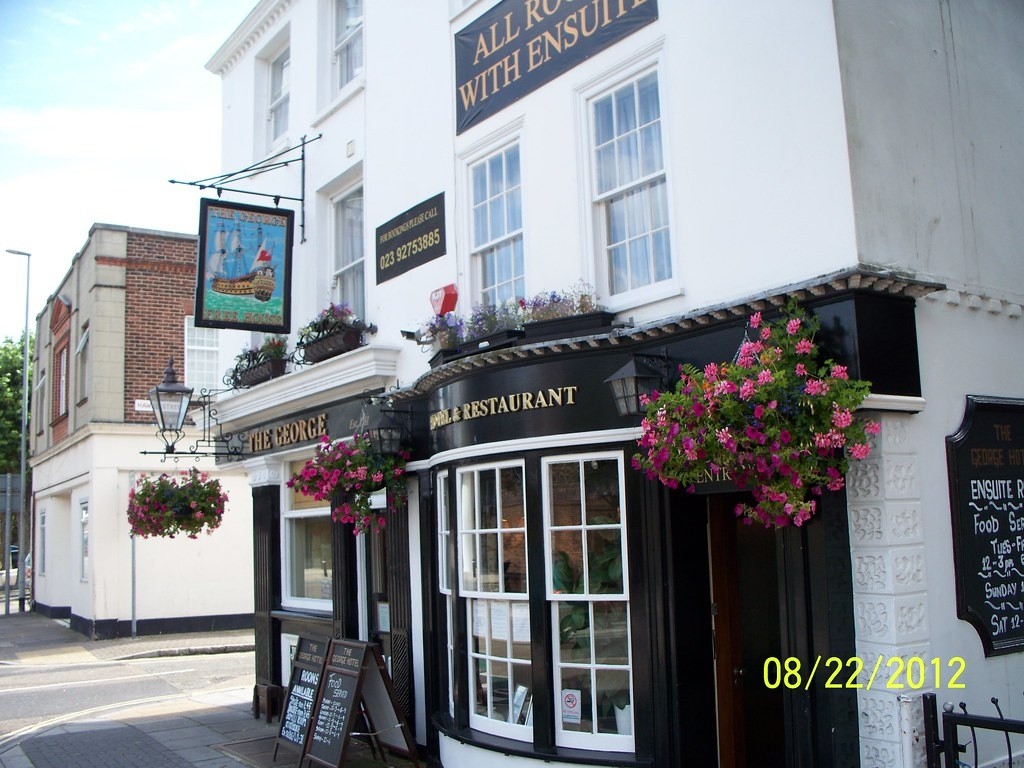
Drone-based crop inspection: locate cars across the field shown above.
[0,545,31,608]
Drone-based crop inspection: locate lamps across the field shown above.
[375,405,417,455]
[140,356,243,462]
[604,347,674,417]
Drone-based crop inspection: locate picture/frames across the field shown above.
[194,199,295,335]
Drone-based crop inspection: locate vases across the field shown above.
[295,327,364,365]
[428,348,463,369]
[458,329,526,356]
[239,358,288,386]
[522,310,624,344]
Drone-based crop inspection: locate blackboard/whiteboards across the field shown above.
[304,638,418,768]
[275,635,339,753]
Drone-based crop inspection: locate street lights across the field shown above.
[5,250,32,614]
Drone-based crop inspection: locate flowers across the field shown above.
[126,466,231,539]
[424,280,608,352]
[231,335,291,389]
[298,302,378,342]
[287,430,414,535]
[633,295,885,530]
